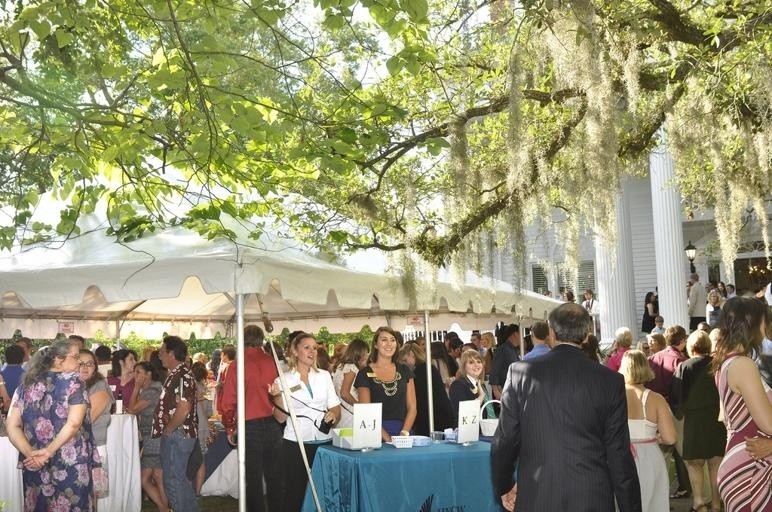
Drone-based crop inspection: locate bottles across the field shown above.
[116,391,123,414]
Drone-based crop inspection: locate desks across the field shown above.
[200,430,239,500]
[301,435,520,512]
[0,413,141,512]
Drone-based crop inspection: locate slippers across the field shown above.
[689,504,707,512]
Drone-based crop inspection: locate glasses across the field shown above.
[81,361,95,367]
[67,354,81,360]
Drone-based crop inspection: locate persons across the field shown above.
[544,291,553,299]
[600,326,634,370]
[724,285,736,301]
[617,348,679,511]
[635,340,653,359]
[192,352,209,366]
[751,278,767,304]
[449,340,467,368]
[92,346,114,385]
[750,305,772,388]
[581,333,603,367]
[519,332,534,356]
[645,324,689,419]
[673,327,728,512]
[689,272,708,332]
[6,337,102,512]
[715,282,727,299]
[698,322,711,337]
[0,375,11,438]
[580,289,600,338]
[398,343,449,439]
[129,361,174,512]
[470,332,487,368]
[640,291,658,335]
[439,348,496,434]
[481,332,497,382]
[704,289,726,326]
[267,332,341,512]
[488,322,522,418]
[564,292,576,304]
[2,346,27,402]
[149,350,170,387]
[520,322,554,361]
[332,338,371,429]
[15,338,34,372]
[646,333,669,358]
[415,337,427,352]
[189,360,211,499]
[104,349,140,414]
[220,324,284,511]
[152,335,200,512]
[703,282,716,295]
[459,343,478,357]
[489,303,642,512]
[713,296,772,512]
[353,326,417,444]
[429,342,462,403]
[744,436,772,461]
[443,332,459,351]
[74,348,115,512]
[202,349,222,419]
[262,339,288,375]
[649,313,667,333]
[215,346,236,429]
[557,287,566,303]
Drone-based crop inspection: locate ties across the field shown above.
[477,384,497,419]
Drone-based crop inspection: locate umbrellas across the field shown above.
[0,170,582,512]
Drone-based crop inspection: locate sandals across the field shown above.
[670,490,690,498]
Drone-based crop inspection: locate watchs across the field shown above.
[401,429,409,437]
